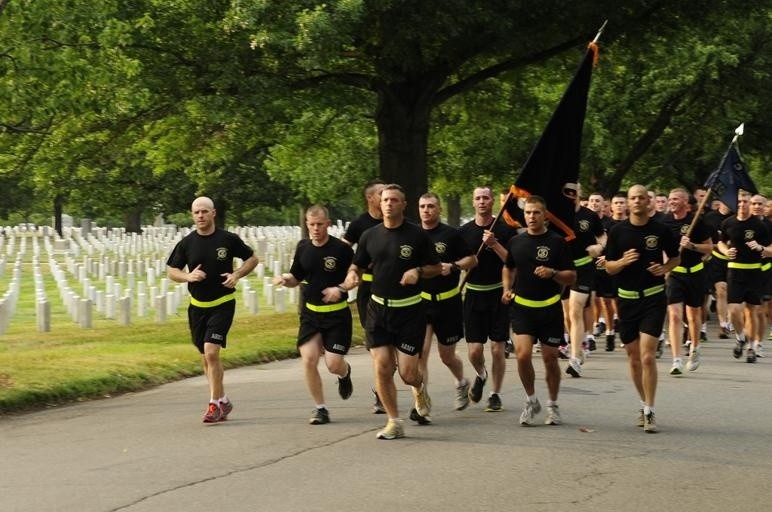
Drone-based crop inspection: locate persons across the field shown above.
[167,196,259,422]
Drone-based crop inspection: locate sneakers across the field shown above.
[377,416,406,440]
[519,397,542,427]
[338,362,353,400]
[202,396,234,423]
[637,403,658,432]
[455,377,470,413]
[544,400,562,425]
[484,391,506,413]
[306,407,331,425]
[370,386,388,414]
[410,383,433,426]
[469,371,488,405]
[498,315,766,378]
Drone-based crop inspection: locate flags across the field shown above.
[503,44,598,243]
[706,138,758,213]
